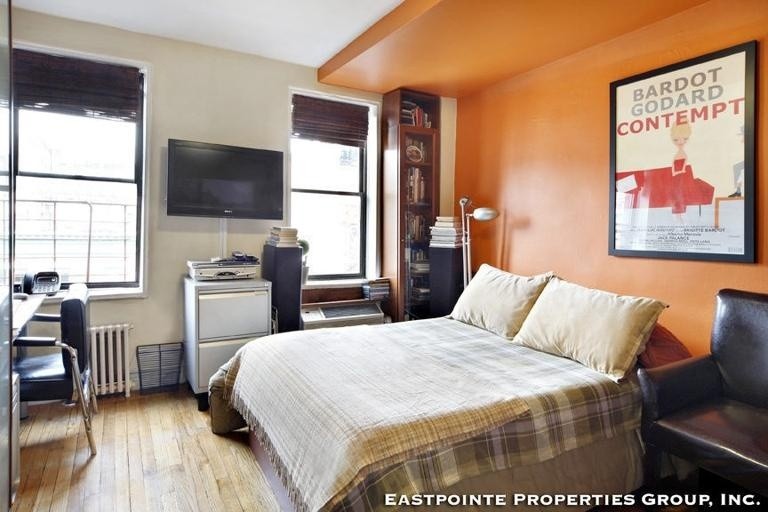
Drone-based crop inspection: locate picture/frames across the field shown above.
[609,39,759,264]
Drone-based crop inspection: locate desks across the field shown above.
[11,294,47,342]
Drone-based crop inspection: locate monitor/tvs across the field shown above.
[167,139,283,220]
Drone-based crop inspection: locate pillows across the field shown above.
[640,320,693,368]
[444,263,555,341]
[508,276,670,385]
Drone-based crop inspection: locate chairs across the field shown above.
[12,283,100,455]
[637,288,767,512]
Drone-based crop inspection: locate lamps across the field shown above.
[460,195,498,290]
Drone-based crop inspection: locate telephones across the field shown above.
[23,272,62,294]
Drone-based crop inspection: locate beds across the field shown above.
[208,316,693,512]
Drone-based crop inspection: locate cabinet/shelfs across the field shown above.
[382,87,441,322]
[184,278,272,412]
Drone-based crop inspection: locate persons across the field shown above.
[727,125,744,198]
[668,118,694,226]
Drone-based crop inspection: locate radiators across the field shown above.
[90,324,134,398]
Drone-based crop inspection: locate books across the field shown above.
[401,99,465,298]
[263,225,298,248]
[361,277,391,300]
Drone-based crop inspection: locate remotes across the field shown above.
[232,251,243,257]
